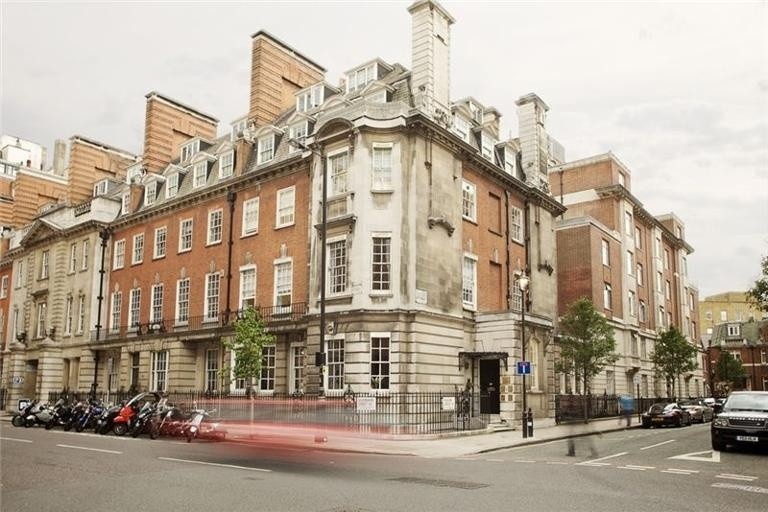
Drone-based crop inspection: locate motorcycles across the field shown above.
[11,391,218,443]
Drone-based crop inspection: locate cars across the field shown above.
[642,398,726,430]
[711,391,768,457]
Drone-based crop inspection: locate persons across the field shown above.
[560,378,600,461]
[617,390,635,429]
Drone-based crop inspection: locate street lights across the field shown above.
[513,269,533,440]
[287,137,327,398]
